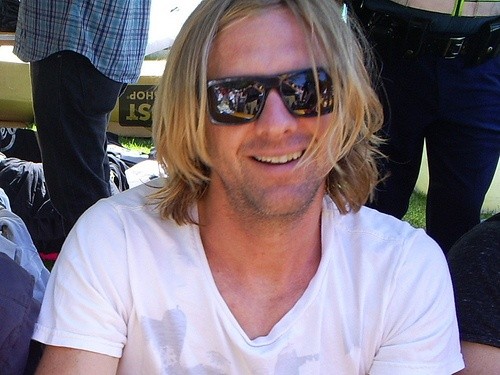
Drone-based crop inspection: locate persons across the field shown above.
[282,70,332,107]
[31,0,466,375]
[12,0,150,239]
[446,213,500,375]
[214,80,264,115]
[333,0,500,252]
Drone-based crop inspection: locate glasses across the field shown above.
[204,67,334,126]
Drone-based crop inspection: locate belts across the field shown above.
[352,0,499,60]
